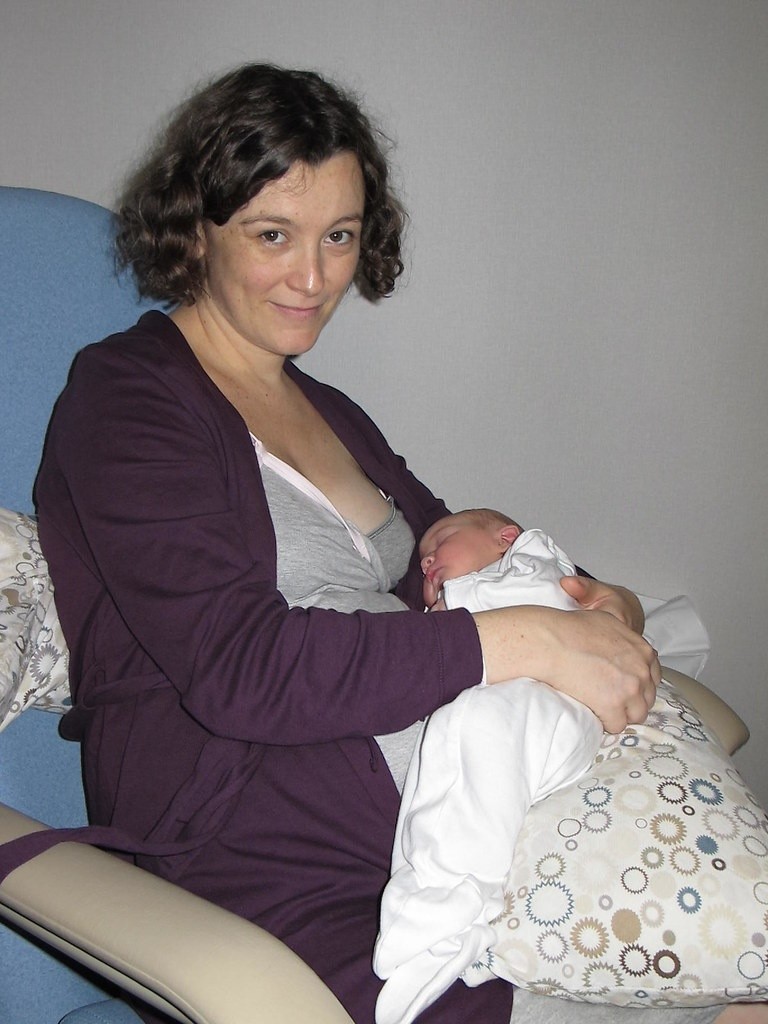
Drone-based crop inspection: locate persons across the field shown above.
[419,508,601,801]
[32,62,768,1024]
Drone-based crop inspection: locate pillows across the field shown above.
[457,676,768,1008]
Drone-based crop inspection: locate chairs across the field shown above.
[0,185,751,1024]
